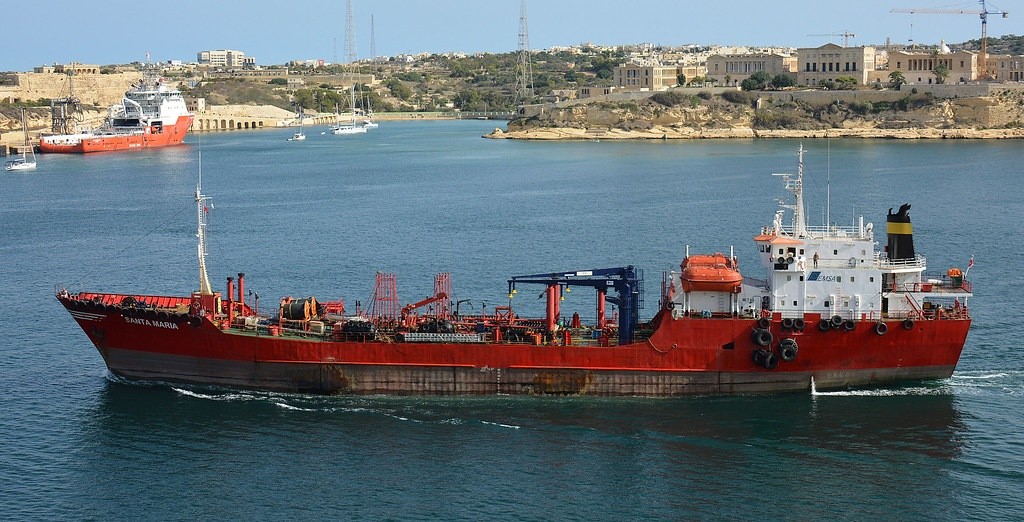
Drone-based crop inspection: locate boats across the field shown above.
[56,138,974,396]
[40,49,195,153]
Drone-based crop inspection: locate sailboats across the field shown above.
[285,105,306,142]
[5,109,37,172]
[329,84,378,135]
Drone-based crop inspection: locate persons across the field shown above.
[813,252,819,269]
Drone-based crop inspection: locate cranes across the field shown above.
[889,0,1008,80]
[807,29,854,46]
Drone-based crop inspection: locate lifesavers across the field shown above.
[751,315,915,370]
[69,299,203,327]
